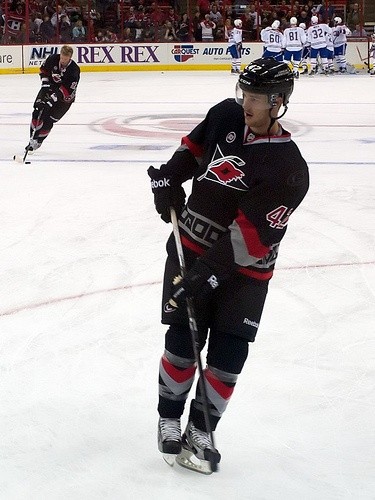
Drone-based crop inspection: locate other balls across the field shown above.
[24,162,31,164]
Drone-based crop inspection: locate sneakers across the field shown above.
[174,421,221,475]
[24,136,42,155]
[157,417,182,465]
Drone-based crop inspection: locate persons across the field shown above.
[150,57,310,474]
[25,46,80,154]
[227,19,244,76]
[261,15,351,78]
[0,0,367,43]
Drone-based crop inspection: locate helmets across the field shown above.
[299,23,307,29]
[271,20,281,30]
[233,19,243,26]
[334,17,342,24]
[311,16,318,24]
[289,17,297,25]
[234,58,294,107]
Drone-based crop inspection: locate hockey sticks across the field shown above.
[13,108,43,163]
[168,206,219,474]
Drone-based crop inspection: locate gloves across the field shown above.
[170,261,215,307]
[148,164,186,223]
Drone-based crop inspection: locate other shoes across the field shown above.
[293,71,299,79]
[300,66,308,74]
[231,65,244,75]
[337,66,347,75]
[309,65,334,75]
